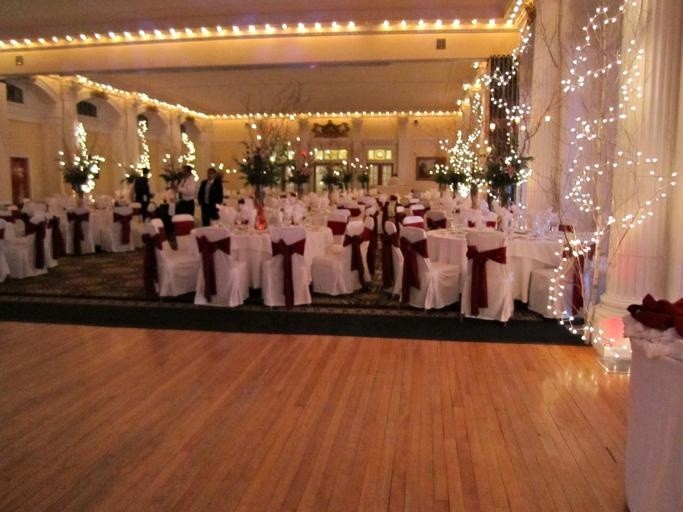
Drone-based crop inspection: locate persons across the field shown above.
[133,168,157,222]
[171,164,197,217]
[197,167,224,227]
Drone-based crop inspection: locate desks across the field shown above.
[623,314,683,512]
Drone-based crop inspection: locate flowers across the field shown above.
[58,138,100,189]
[435,152,535,189]
[237,81,369,188]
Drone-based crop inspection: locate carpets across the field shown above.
[597,358,631,373]
[0,246,581,348]
